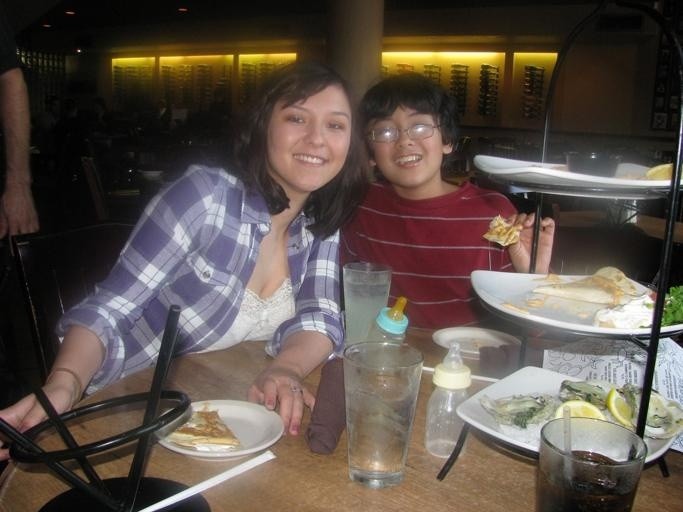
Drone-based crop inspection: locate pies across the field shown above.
[483,216,523,248]
[170,409,239,447]
[532,266,643,307]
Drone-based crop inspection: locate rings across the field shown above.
[290,385,300,391]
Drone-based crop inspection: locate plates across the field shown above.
[432,325,525,353]
[151,398,285,463]
[455,366,683,471]
[472,153,682,204]
[470,270,682,337]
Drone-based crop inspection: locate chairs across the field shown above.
[12,216,139,389]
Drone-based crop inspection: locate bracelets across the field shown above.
[44,367,81,405]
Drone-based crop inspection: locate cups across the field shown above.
[340,260,393,341]
[342,340,423,487]
[563,140,625,177]
[535,418,650,510]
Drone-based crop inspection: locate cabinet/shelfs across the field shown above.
[109,55,157,145]
[511,52,560,130]
[155,56,235,151]
[237,54,295,117]
[381,49,504,127]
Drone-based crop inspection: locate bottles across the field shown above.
[110,64,286,110]
[360,294,408,365]
[449,65,546,121]
[382,65,441,82]
[127,168,134,185]
[420,343,476,461]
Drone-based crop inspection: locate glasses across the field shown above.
[365,124,441,143]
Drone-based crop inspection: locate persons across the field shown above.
[34,83,236,188]
[0,62,372,458]
[1,0,42,264]
[335,71,556,332]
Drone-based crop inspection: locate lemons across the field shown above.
[556,385,631,427]
[645,163,674,183]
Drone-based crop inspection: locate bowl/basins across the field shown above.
[135,168,165,184]
